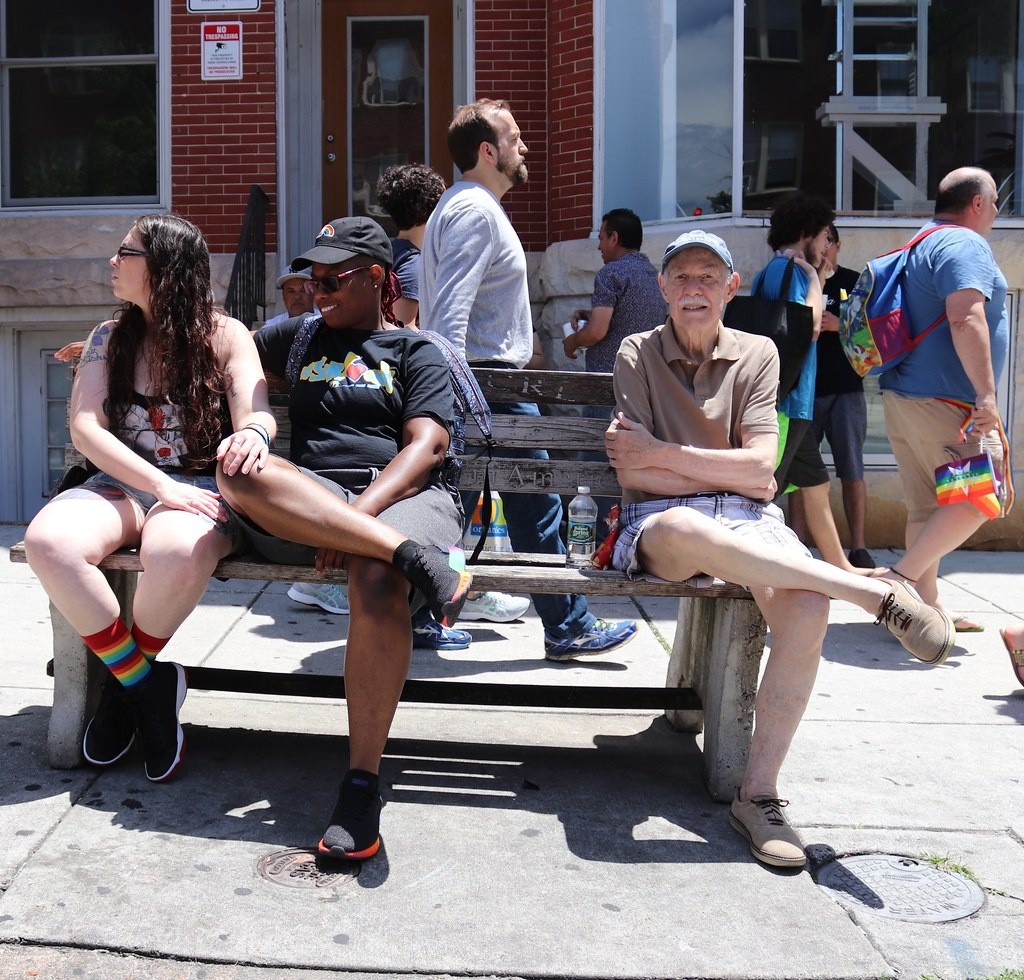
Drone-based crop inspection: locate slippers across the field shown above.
[867,568,879,577]
[999,628,1024,686]
[953,616,984,632]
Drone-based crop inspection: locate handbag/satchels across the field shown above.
[722,255,814,399]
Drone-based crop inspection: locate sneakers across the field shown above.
[406,544,473,628]
[848,549,876,568]
[83,671,135,767]
[873,577,956,665]
[729,785,807,866]
[544,618,639,660]
[131,661,188,783]
[286,582,349,615]
[458,592,531,622]
[319,785,383,858]
[412,620,473,649]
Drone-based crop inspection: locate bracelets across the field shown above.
[242,423,270,447]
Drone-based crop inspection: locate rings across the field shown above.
[233,440,242,446]
[315,555,324,560]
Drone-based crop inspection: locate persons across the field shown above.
[605,230,954,866]
[749,196,890,578]
[408,97,637,663]
[562,209,668,550]
[1001,628,1024,685]
[260,266,320,328]
[53,216,474,859]
[877,167,1008,632]
[25,215,278,781]
[287,164,531,623]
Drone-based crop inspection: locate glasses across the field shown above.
[828,237,837,245]
[118,246,149,260]
[301,265,370,296]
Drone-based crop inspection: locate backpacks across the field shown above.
[839,224,974,377]
[283,314,497,488]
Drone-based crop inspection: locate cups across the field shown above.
[561,320,588,354]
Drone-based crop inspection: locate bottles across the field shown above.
[567,487,598,567]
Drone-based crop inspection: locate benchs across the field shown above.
[11,346,769,803]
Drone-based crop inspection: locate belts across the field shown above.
[468,361,517,369]
[645,490,735,501]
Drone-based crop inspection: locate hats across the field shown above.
[661,230,734,273]
[291,216,393,271]
[276,265,312,289]
